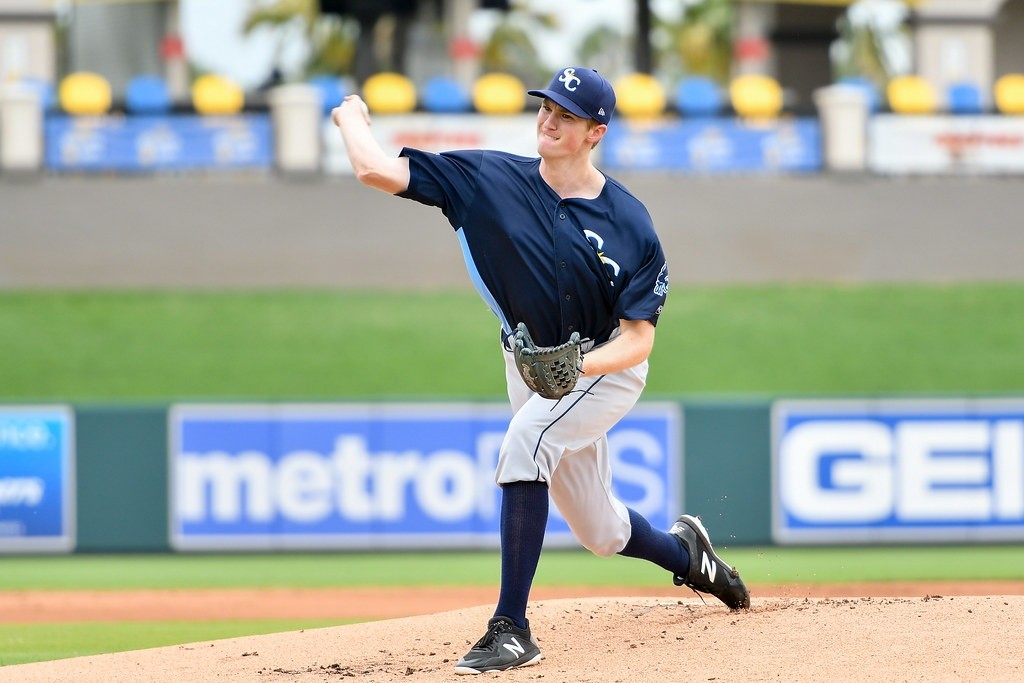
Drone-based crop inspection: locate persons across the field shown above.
[332,66,750,674]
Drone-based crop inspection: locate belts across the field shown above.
[501,329,610,349]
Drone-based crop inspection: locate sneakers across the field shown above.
[455,616,541,674]
[667,515,750,610]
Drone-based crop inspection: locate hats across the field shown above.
[527,65,617,126]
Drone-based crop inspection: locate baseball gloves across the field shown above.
[511,320,586,401]
